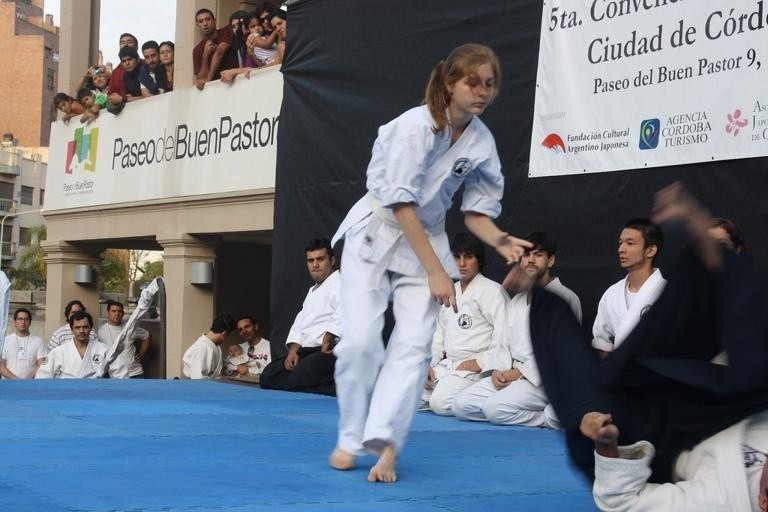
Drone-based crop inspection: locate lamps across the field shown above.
[191,261,213,284]
[75,265,93,283]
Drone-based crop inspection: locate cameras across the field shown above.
[93,69,102,76]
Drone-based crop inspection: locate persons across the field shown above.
[704,214,744,262]
[451,227,583,427]
[224,315,272,379]
[53,9,287,125]
[501,180,768,511]
[224,345,261,377]
[414,234,510,417]
[181,313,237,379]
[328,43,534,482]
[259,239,340,397]
[1,297,152,378]
[542,216,671,433]
